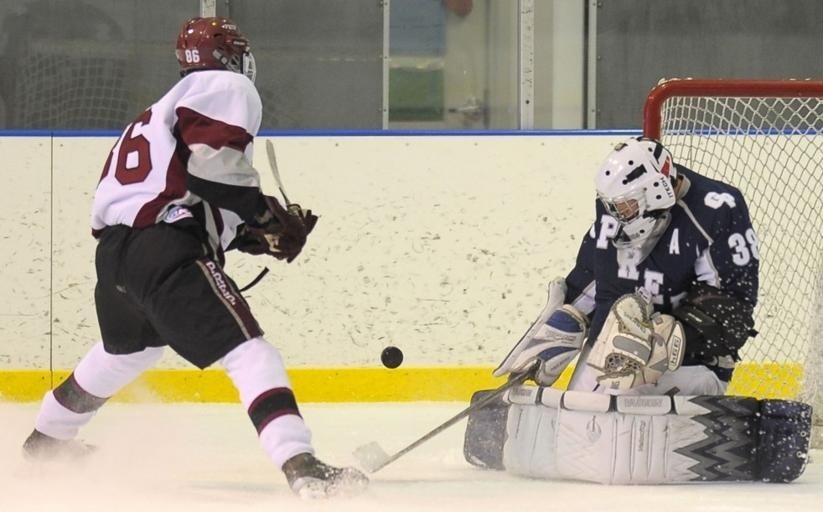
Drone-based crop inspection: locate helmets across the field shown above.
[594,133,677,247]
[175,15,258,87]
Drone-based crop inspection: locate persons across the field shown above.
[484,135,765,403]
[21,14,371,496]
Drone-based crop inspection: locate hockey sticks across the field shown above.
[351,362,539,474]
[265,140,290,205]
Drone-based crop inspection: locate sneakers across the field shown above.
[20,428,99,466]
[280,451,371,503]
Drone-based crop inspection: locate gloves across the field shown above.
[239,194,318,264]
